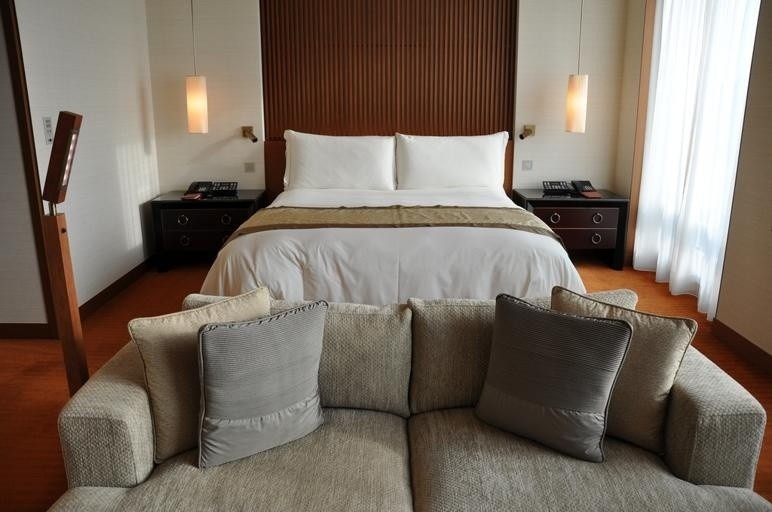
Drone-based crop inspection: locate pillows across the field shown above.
[282,129,395,192]
[474,293,634,464]
[198,300,329,467]
[128,286,272,464]
[396,130,510,192]
[551,285,698,454]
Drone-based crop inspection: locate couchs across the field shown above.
[47,288,772,511]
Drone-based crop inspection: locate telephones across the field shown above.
[571,181,597,192]
[184,181,212,199]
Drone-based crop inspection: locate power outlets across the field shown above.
[244,162,255,174]
[521,159,533,171]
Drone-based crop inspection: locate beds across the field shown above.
[199,129,588,306]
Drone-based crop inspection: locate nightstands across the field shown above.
[150,188,266,274]
[513,187,631,272]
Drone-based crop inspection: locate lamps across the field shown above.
[185,0,210,134]
[39,110,90,397]
[563,0,589,135]
